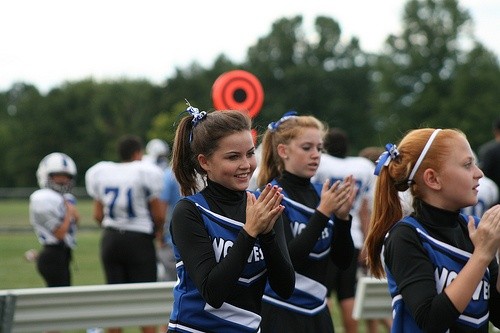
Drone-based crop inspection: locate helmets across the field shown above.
[145,138,172,169]
[37,152,77,189]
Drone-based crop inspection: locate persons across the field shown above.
[85,135,164,333]
[141,138,184,282]
[167,106,295,333]
[26,152,81,286]
[364,128,500,333]
[250,112,358,333]
[308,128,412,333]
[463,119,500,221]
